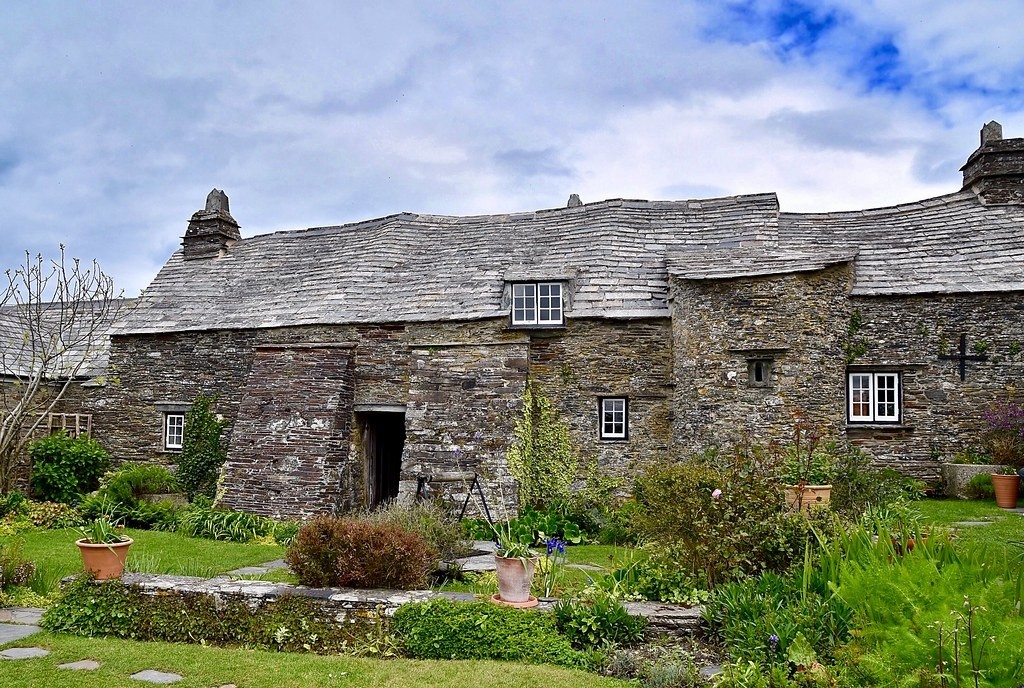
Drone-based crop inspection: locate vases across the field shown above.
[495,553,539,603]
[75,538,134,581]
[992,474,1020,508]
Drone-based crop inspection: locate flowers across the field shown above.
[491,524,545,589]
[78,492,130,556]
[995,465,1018,476]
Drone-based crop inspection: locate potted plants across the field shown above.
[781,450,834,518]
